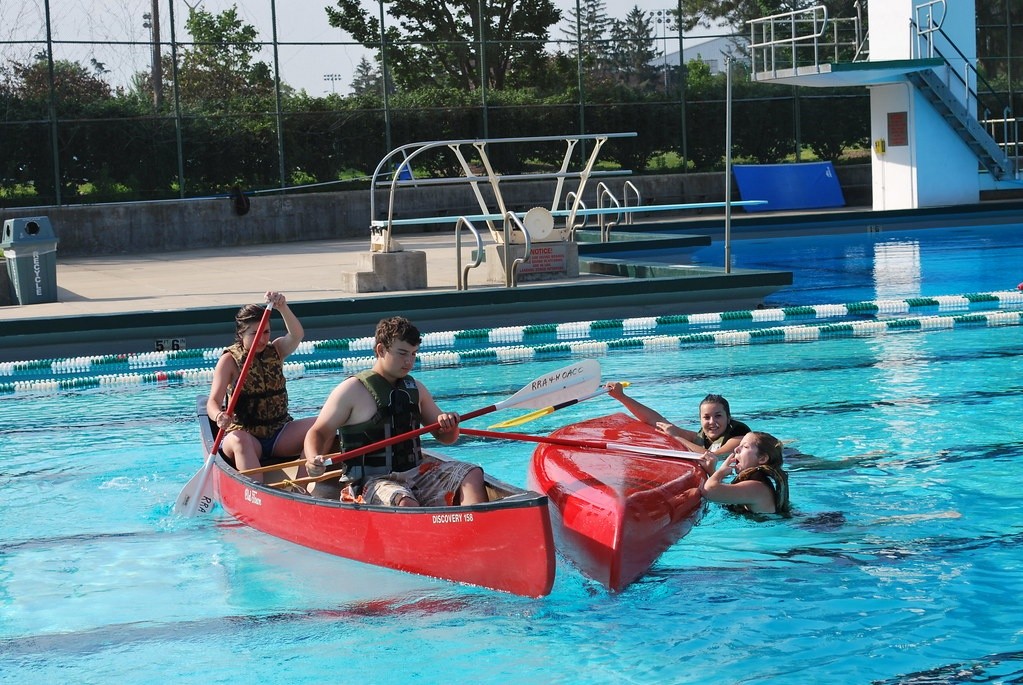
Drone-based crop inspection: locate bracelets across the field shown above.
[213,411,223,422]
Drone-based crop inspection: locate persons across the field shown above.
[207,288,335,499]
[604,374,905,489]
[697,431,968,555]
[300,316,495,526]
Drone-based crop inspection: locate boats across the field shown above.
[525,409,708,594]
[193,392,555,599]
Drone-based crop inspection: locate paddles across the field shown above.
[317,359,706,466]
[174,301,274,519]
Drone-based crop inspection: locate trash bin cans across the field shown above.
[0,216,60,306]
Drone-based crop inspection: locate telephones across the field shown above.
[874,139,885,154]
